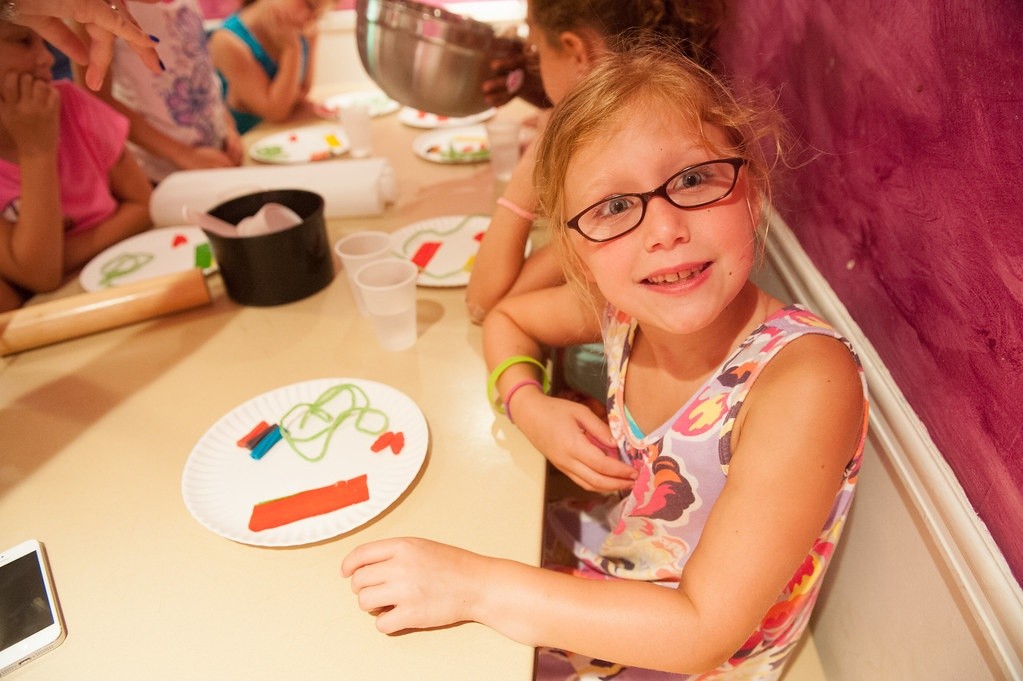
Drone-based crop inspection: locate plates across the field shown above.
[248,123,351,162]
[77,223,223,292]
[412,123,508,164]
[181,379,429,549]
[394,213,533,288]
[398,101,498,129]
[311,89,403,120]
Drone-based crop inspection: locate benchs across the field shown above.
[744,194,1023,681]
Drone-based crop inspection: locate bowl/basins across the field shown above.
[356,0,529,118]
[199,189,334,305]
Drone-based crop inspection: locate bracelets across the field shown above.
[487,355,550,415]
[505,379,544,424]
[497,197,535,220]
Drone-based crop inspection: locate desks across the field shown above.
[0,100,558,681]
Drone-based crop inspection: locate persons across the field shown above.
[0,0,166,91]
[66,0,242,189]
[466,0,719,325]
[343,47,870,681]
[206,0,339,136]
[482,37,554,110]
[0,0,154,316]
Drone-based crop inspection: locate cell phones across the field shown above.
[0,540,67,678]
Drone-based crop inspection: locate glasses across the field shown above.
[566,158,748,243]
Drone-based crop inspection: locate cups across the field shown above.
[354,260,418,350]
[334,230,396,313]
